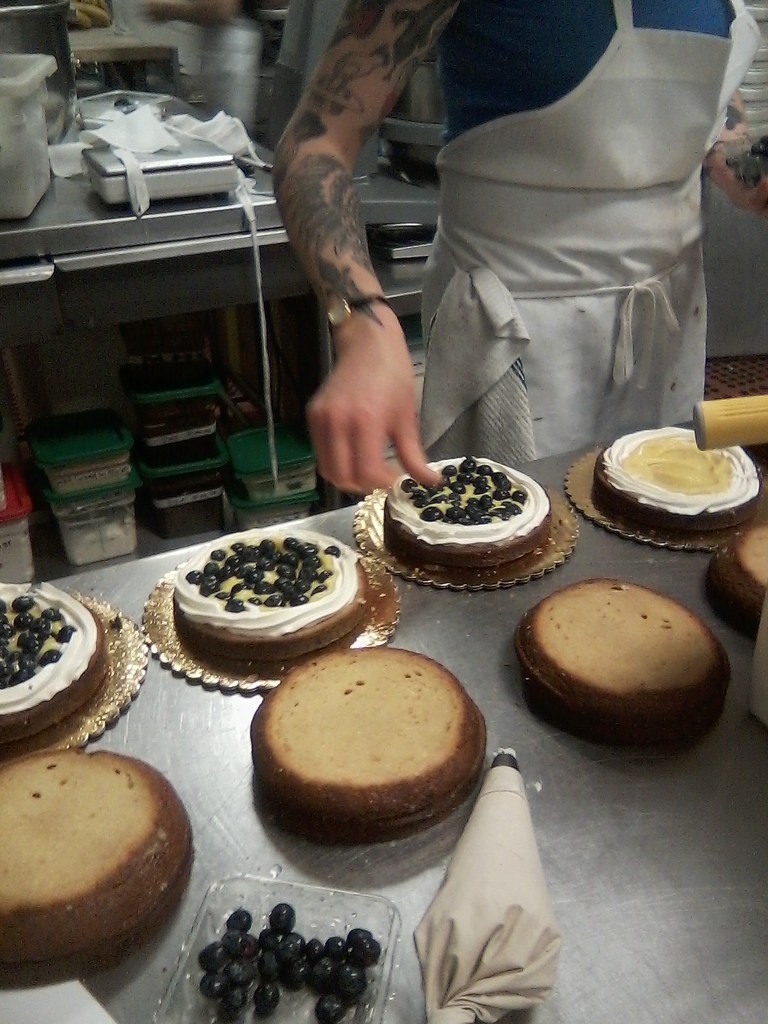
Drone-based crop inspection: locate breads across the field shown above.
[514,577,731,747]
[249,645,486,848]
[704,520,768,639]
[67,0,112,29]
[0,746,193,989]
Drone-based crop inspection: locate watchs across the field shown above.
[326,295,406,337]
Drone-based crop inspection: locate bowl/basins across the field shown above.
[155,872,402,1024]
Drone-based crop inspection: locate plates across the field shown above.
[562,446,768,552]
[142,557,402,693]
[0,588,149,767]
[353,487,581,593]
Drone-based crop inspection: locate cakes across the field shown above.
[171,529,372,680]
[383,453,552,585]
[591,426,762,546]
[0,578,111,762]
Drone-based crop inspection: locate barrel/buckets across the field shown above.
[0,0,80,144]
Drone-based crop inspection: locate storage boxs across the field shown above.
[0,342,319,586]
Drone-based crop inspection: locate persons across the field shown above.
[112,0,260,136]
[273,0,768,494]
